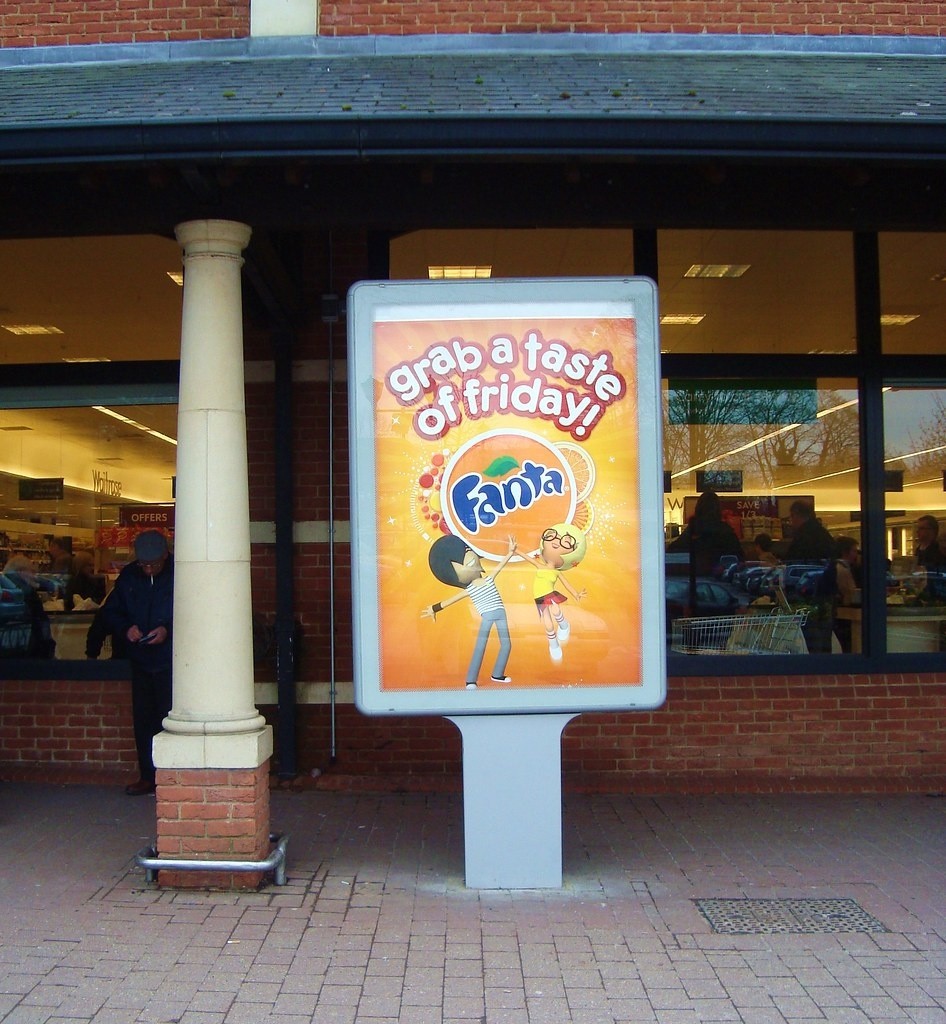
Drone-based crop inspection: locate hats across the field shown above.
[134,530,167,561]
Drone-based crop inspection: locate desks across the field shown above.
[837,603,946,654]
[46,610,113,660]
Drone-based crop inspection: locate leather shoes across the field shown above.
[126,778,155,795]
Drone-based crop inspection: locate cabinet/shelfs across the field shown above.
[0,547,76,565]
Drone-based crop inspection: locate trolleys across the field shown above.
[671,606,818,655]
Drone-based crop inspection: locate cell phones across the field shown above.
[138,633,157,645]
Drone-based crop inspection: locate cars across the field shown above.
[34,573,70,601]
[665,577,759,616]
[0,571,26,619]
[718,555,828,598]
[903,572,946,596]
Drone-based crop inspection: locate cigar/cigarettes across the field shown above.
[151,574,153,585]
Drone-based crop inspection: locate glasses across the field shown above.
[137,563,160,568]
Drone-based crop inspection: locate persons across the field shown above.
[833,536,863,654]
[50,537,74,574]
[85,528,174,794]
[754,533,781,566]
[668,492,747,617]
[0,554,57,661]
[786,501,838,654]
[64,551,107,611]
[914,515,946,605]
